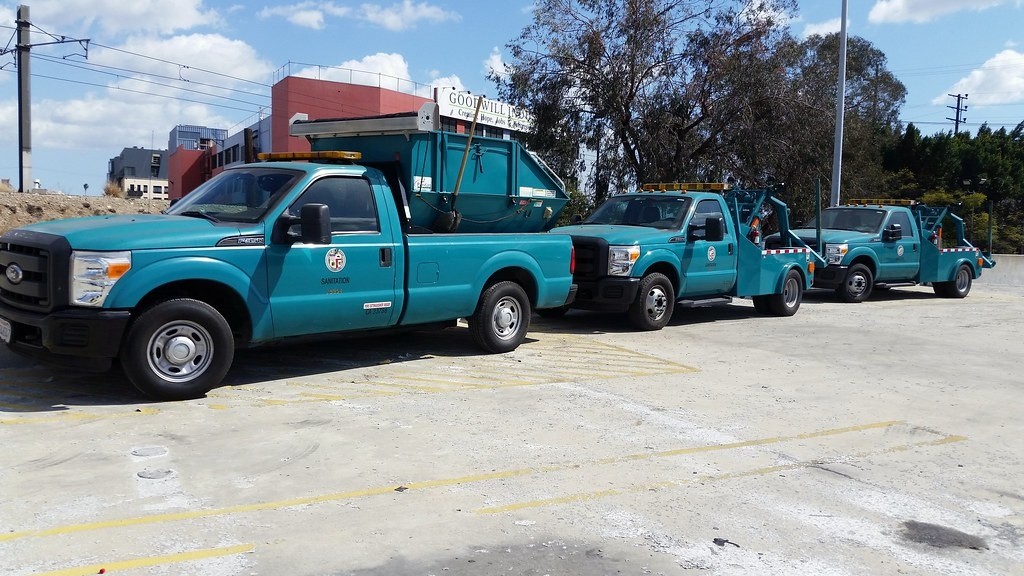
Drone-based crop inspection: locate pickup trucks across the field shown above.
[764,197,996,303]
[549,182,830,332]
[0,102,578,402]
